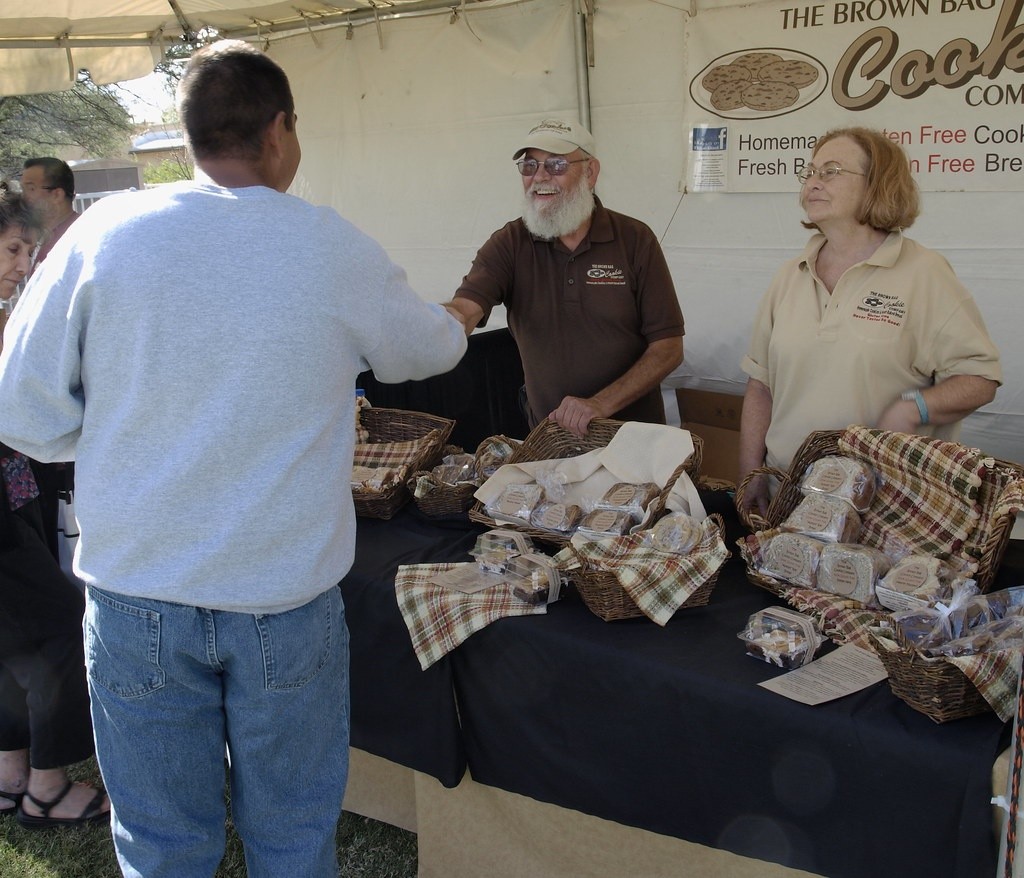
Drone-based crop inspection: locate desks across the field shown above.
[334,503,416,833]
[416,505,1024,878]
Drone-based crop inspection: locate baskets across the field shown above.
[351,407,457,520]
[547,511,733,622]
[468,405,706,547]
[868,584,1023,725]
[406,444,484,518]
[737,424,1023,634]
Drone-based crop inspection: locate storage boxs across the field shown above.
[353,406,1023,724]
[675,388,745,485]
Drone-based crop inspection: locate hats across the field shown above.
[513,119,596,162]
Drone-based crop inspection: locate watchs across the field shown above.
[900,386,929,427]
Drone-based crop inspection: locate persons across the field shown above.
[436,115,687,447]
[16,156,89,308]
[1,40,468,876]
[0,192,125,832]
[737,128,1006,535]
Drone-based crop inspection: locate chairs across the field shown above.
[355,329,531,455]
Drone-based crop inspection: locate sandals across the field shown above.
[16,779,111,830]
[0,790,26,815]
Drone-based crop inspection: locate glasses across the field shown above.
[796,164,866,184]
[516,155,591,177]
